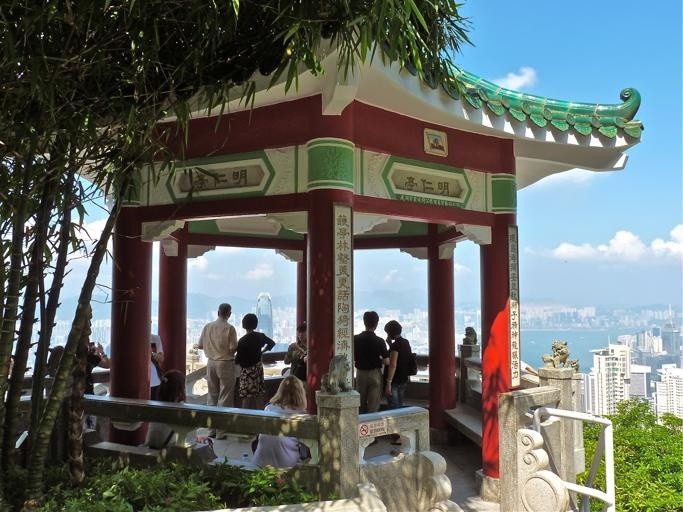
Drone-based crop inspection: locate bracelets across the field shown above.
[385,378,392,383]
[101,354,107,359]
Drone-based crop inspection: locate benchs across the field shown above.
[179,358,491,475]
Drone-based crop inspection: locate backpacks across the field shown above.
[407,352,417,375]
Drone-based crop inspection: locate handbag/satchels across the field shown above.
[299,444,311,459]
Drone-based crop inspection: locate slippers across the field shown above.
[390,440,401,445]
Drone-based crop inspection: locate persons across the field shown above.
[249,374,307,470]
[353,310,390,416]
[142,369,214,450]
[196,302,238,440]
[381,320,412,409]
[83,338,108,395]
[282,318,307,381]
[146,320,165,398]
[230,313,275,410]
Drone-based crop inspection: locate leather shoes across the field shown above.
[209,433,227,440]
